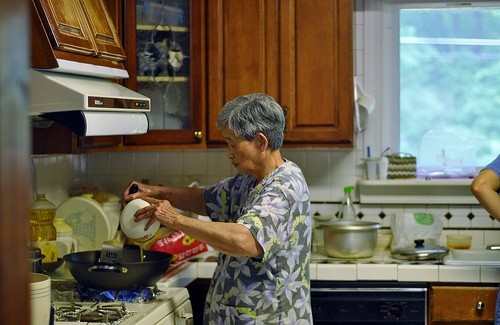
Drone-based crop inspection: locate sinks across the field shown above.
[442,247,500,268]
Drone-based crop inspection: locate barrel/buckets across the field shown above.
[30,273,51,325]
[81,194,121,240]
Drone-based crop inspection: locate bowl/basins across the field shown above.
[446,236,472,249]
[375,234,392,251]
[42,257,64,272]
[120,198,160,243]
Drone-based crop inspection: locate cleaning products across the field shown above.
[337,185,356,220]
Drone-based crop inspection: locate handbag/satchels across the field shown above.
[386,152,417,177]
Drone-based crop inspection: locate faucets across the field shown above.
[486,245,500,250]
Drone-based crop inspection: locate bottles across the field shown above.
[29,192,58,262]
[312,215,334,257]
[337,186,356,221]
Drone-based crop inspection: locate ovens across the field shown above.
[310,281,428,325]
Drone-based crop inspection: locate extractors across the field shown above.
[25,68,152,138]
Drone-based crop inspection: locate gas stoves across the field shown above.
[51,280,194,325]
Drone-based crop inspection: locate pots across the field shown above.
[62,244,174,292]
[318,220,384,260]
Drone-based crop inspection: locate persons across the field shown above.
[121,92,313,325]
[470,153,500,325]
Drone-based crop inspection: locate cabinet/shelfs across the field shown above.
[428,286,499,325]
[33,0,206,154]
[206,0,354,149]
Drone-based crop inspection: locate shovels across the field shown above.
[99,182,139,263]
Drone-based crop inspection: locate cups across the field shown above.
[443,148,476,178]
[361,158,383,180]
